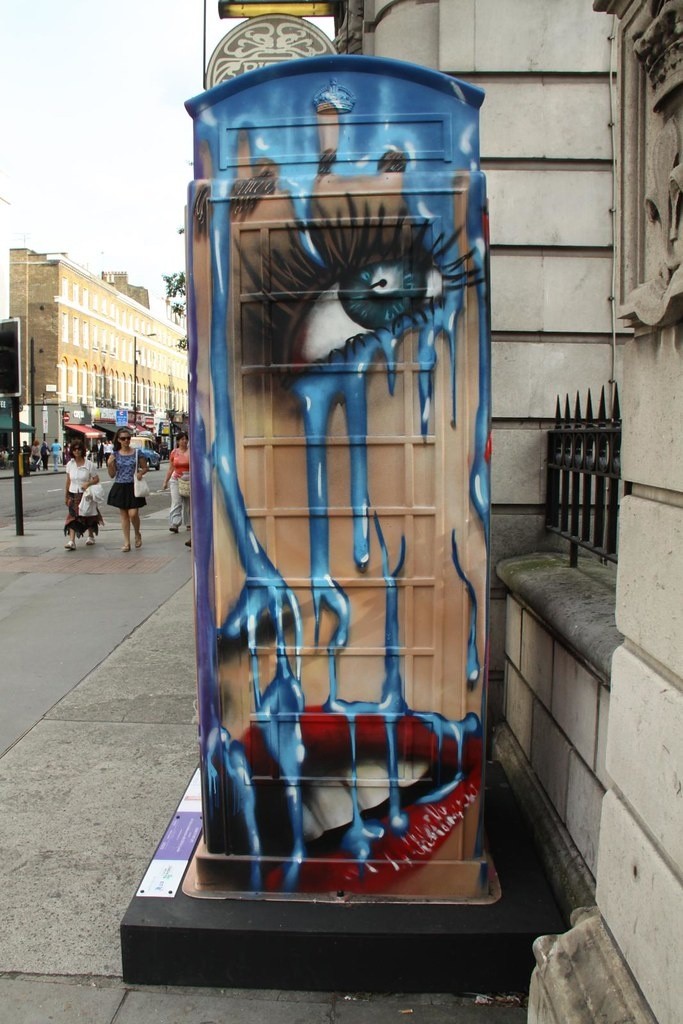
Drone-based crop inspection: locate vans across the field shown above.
[129,436,156,453]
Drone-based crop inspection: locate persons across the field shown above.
[163,432,192,533]
[0,435,169,472]
[107,428,149,552]
[64,438,100,550]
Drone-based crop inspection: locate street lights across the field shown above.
[133,333,157,436]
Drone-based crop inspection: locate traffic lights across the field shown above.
[0,318,20,398]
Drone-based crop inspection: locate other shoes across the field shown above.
[134,534,141,548]
[169,527,178,533]
[121,543,131,552]
[86,536,95,544]
[65,541,76,550]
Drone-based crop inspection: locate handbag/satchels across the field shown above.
[177,477,190,497]
[134,449,150,497]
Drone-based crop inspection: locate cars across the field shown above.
[140,449,162,470]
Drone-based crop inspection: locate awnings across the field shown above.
[126,422,147,433]
[93,421,137,436]
[66,424,106,438]
[0,413,37,432]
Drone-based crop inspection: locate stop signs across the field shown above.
[64,414,70,422]
